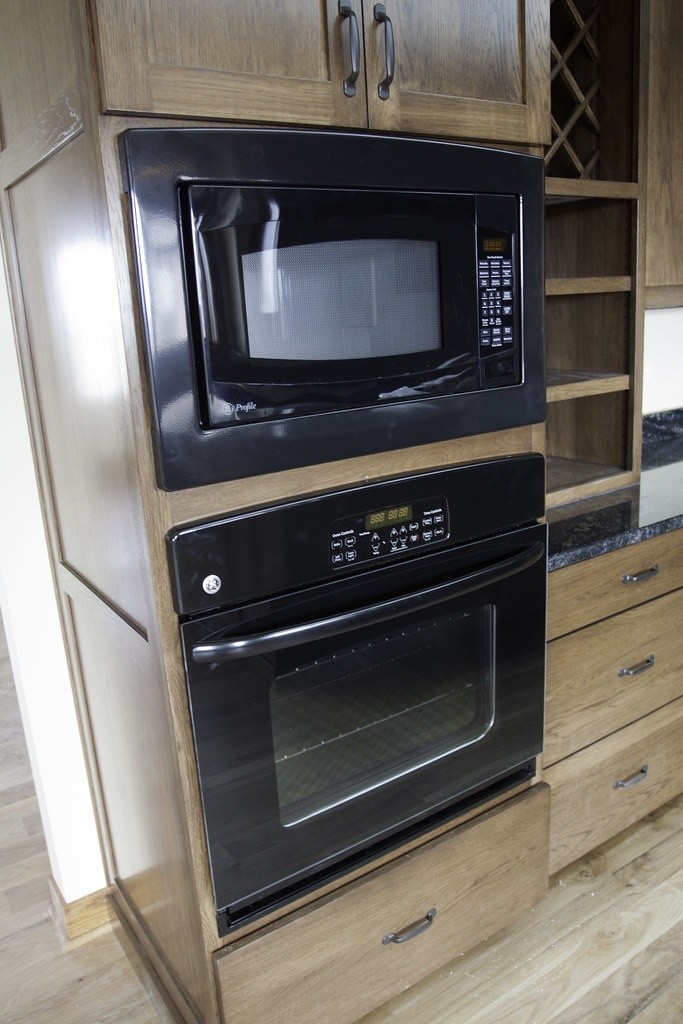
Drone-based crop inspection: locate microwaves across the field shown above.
[116,105,547,491]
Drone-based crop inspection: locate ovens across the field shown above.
[165,451,548,934]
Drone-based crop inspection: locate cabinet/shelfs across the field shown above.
[90,781,550,1024]
[536,529,682,877]
[0,0,549,149]
[598,1,682,310]
[543,176,640,511]
